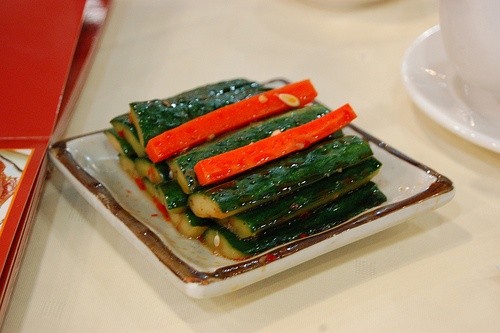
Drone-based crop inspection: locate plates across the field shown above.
[401,23,499,155]
[47,78,455,298]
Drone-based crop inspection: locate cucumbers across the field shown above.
[104,78,387,261]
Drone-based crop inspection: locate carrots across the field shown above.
[145,78,318,162]
[194,102,357,186]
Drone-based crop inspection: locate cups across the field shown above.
[436,0,500,129]
